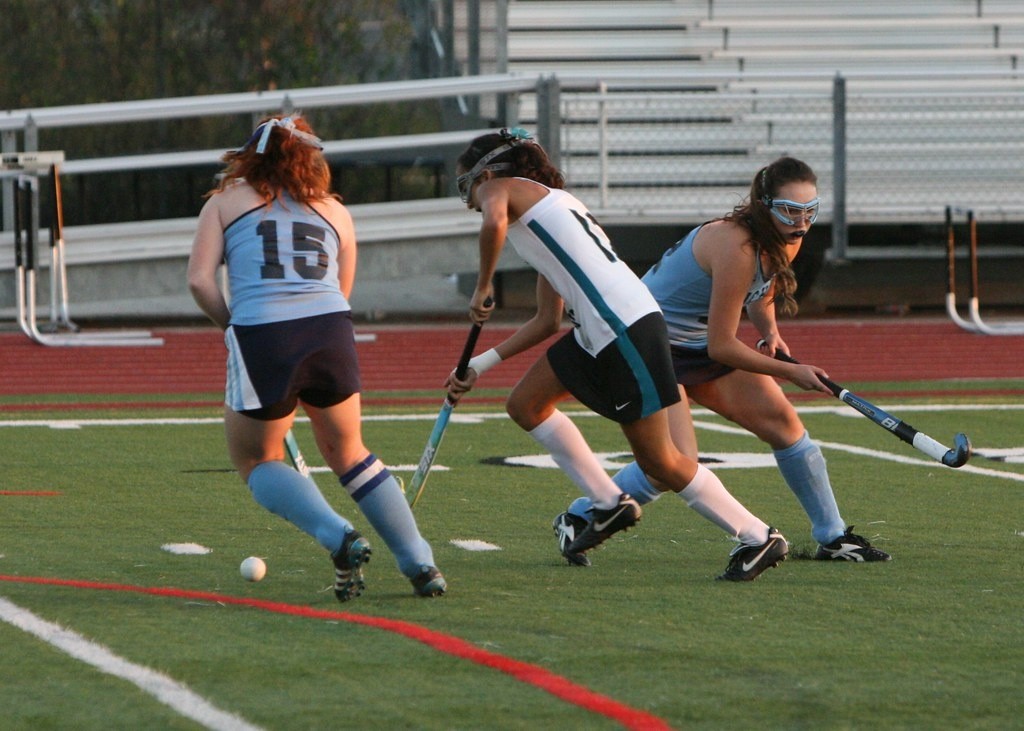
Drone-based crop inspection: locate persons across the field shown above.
[443,127,790,582]
[640,158,895,563]
[185,112,448,604]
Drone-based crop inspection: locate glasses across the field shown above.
[768,198,820,225]
[456,172,474,203]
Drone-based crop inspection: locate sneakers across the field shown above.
[568,492,643,556]
[329,525,372,603]
[409,562,447,599]
[552,510,594,567]
[715,526,790,582]
[815,534,892,562]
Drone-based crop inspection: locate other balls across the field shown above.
[240,556,266,582]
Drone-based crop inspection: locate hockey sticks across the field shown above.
[284,296,493,510]
[755,338,972,468]
